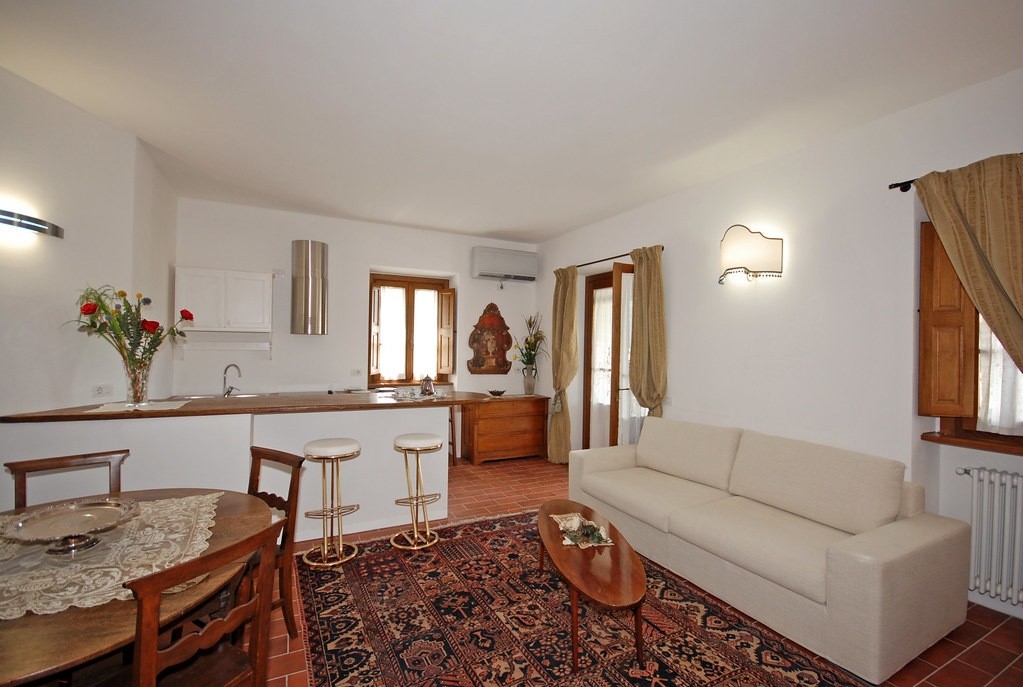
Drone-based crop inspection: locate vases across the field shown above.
[522,364,537,395]
[121,360,151,408]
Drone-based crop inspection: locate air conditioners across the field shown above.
[472,246,538,283]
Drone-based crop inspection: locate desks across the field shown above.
[0,488,273,687]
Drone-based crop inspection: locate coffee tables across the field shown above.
[538,498,647,673]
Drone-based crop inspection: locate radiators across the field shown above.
[955,467,1023,611]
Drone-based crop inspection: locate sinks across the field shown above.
[232,393,262,398]
[185,395,212,399]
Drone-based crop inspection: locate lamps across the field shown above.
[0,210,66,239]
[719,224,784,284]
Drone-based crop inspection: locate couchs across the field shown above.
[568,417,972,684]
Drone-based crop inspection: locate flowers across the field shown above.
[513,314,549,365]
[61,285,194,403]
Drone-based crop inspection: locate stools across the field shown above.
[389,433,444,552]
[305,438,360,566]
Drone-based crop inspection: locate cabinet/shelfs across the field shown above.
[173,267,273,336]
[462,396,550,465]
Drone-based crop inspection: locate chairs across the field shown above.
[246,446,305,641]
[4,449,129,511]
[123,516,288,687]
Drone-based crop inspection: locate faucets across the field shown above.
[223,364,242,398]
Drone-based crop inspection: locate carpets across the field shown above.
[291,511,873,686]
